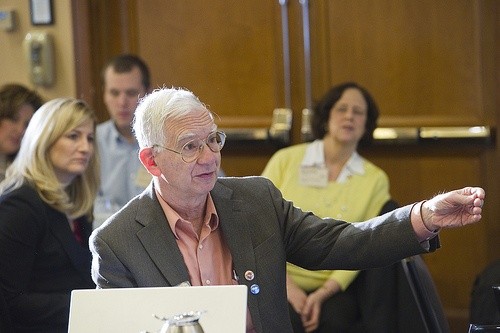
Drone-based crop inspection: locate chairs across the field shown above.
[397,255,452,333]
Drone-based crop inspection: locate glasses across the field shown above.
[147,130,227,165]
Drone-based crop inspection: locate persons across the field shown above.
[89,88,485,333]
[260,82,391,333]
[0,84,44,183]
[91,54,152,231]
[0,98,101,333]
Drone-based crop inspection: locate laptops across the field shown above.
[68,285,247,333]
